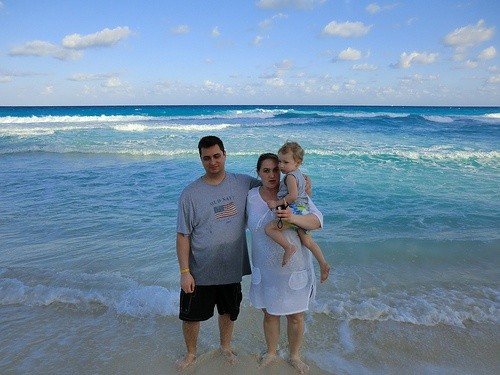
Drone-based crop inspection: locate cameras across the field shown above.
[277,205,286,218]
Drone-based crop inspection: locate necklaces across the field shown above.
[205,176,224,184]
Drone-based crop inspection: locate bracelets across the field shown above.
[181,268,190,273]
[283,197,287,206]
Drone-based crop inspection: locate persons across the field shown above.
[264,142,330,283]
[173,135,312,371]
[245,153,323,373]
